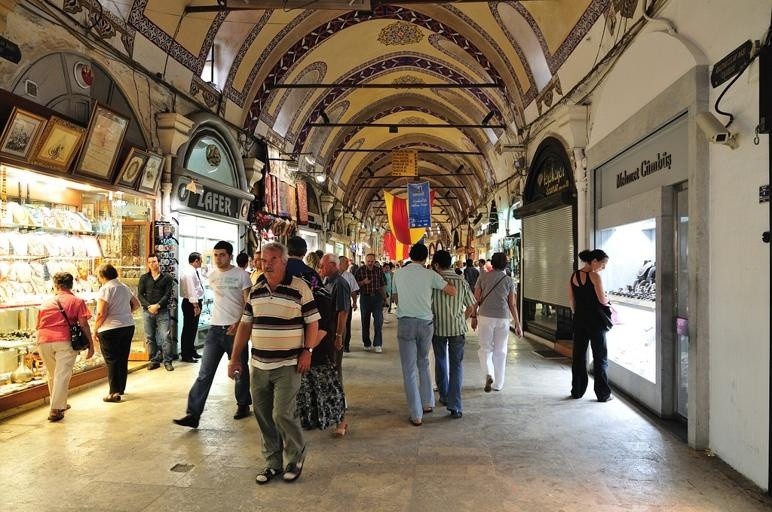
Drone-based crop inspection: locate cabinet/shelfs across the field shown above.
[594,186,675,419]
[0,162,155,419]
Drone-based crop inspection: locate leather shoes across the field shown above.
[165,363,174,372]
[147,363,160,370]
[233,406,251,419]
[193,353,202,358]
[172,415,200,430]
[182,357,198,363]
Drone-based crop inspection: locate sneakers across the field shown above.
[407,416,422,427]
[450,409,463,419]
[364,345,371,352]
[373,346,384,353]
[423,407,433,413]
[484,377,495,394]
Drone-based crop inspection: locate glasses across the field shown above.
[316,263,333,269]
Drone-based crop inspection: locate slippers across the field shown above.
[333,423,348,439]
[47,411,64,421]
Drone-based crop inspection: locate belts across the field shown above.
[209,324,232,332]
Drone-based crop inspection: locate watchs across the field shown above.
[303,346,313,354]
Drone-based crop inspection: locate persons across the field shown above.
[172,242,253,429]
[454,260,463,276]
[354,254,387,353]
[382,263,392,323]
[283,275,348,440]
[432,250,478,419]
[479,259,488,274]
[338,255,360,352]
[136,253,175,371]
[286,236,324,294]
[93,263,140,402]
[34,272,96,420]
[236,253,248,271]
[392,242,457,426]
[566,249,614,403]
[178,252,205,363]
[229,243,321,485]
[468,252,522,392]
[248,251,263,287]
[319,253,350,388]
[463,259,479,294]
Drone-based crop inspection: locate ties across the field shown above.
[195,271,204,291]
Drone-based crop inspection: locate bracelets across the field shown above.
[335,333,343,339]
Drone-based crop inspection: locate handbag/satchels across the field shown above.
[53,297,90,352]
[574,270,614,331]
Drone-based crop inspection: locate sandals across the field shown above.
[281,458,306,482]
[103,392,120,402]
[254,466,284,484]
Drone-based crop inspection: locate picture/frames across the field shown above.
[0,107,48,166]
[135,151,166,196]
[31,115,88,174]
[72,100,131,183]
[112,146,151,189]
